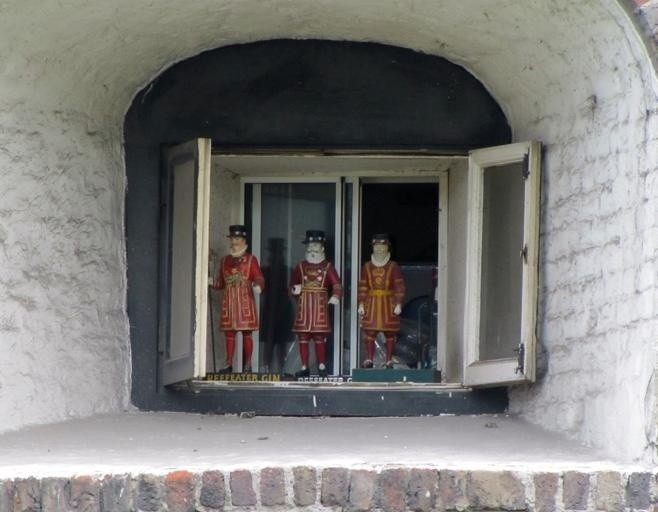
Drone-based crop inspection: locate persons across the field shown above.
[289,229,343,377]
[207,224,265,373]
[357,232,408,369]
